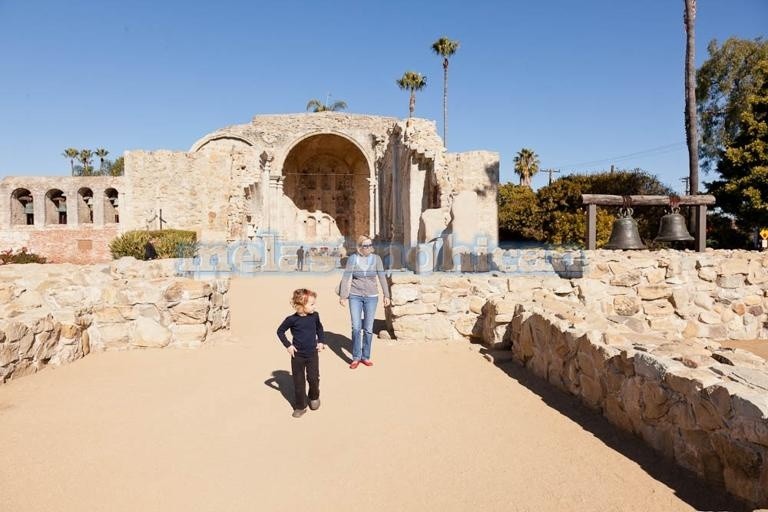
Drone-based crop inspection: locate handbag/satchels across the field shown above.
[335,270,352,299]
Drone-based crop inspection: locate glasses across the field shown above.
[361,244,373,249]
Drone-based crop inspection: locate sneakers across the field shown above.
[350,360,360,369]
[308,397,320,410]
[292,408,306,418]
[361,359,373,366]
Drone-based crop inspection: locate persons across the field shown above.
[143,238,161,261]
[275,288,327,418]
[295,245,305,271]
[340,234,393,370]
